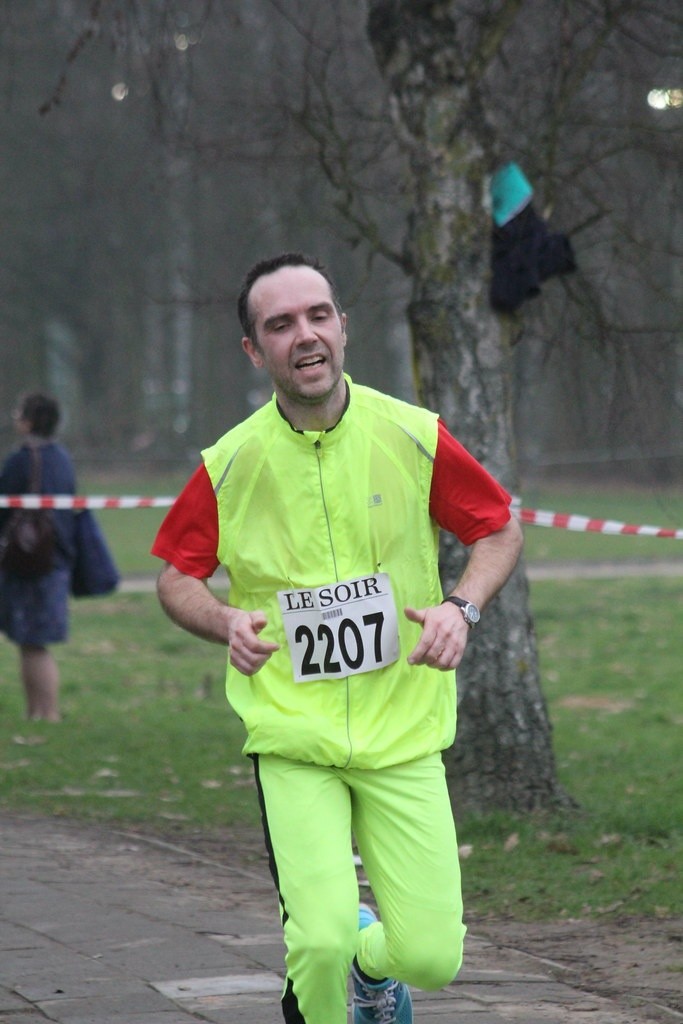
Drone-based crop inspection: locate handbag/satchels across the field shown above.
[71,507,120,598]
[0,506,54,578]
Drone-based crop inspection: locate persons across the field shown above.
[1,394,81,721]
[147,253,523,1024]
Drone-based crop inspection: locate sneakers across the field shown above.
[346,903,413,1024]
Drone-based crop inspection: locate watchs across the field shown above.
[441,596,480,630]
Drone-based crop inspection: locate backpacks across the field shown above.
[489,148,551,311]
